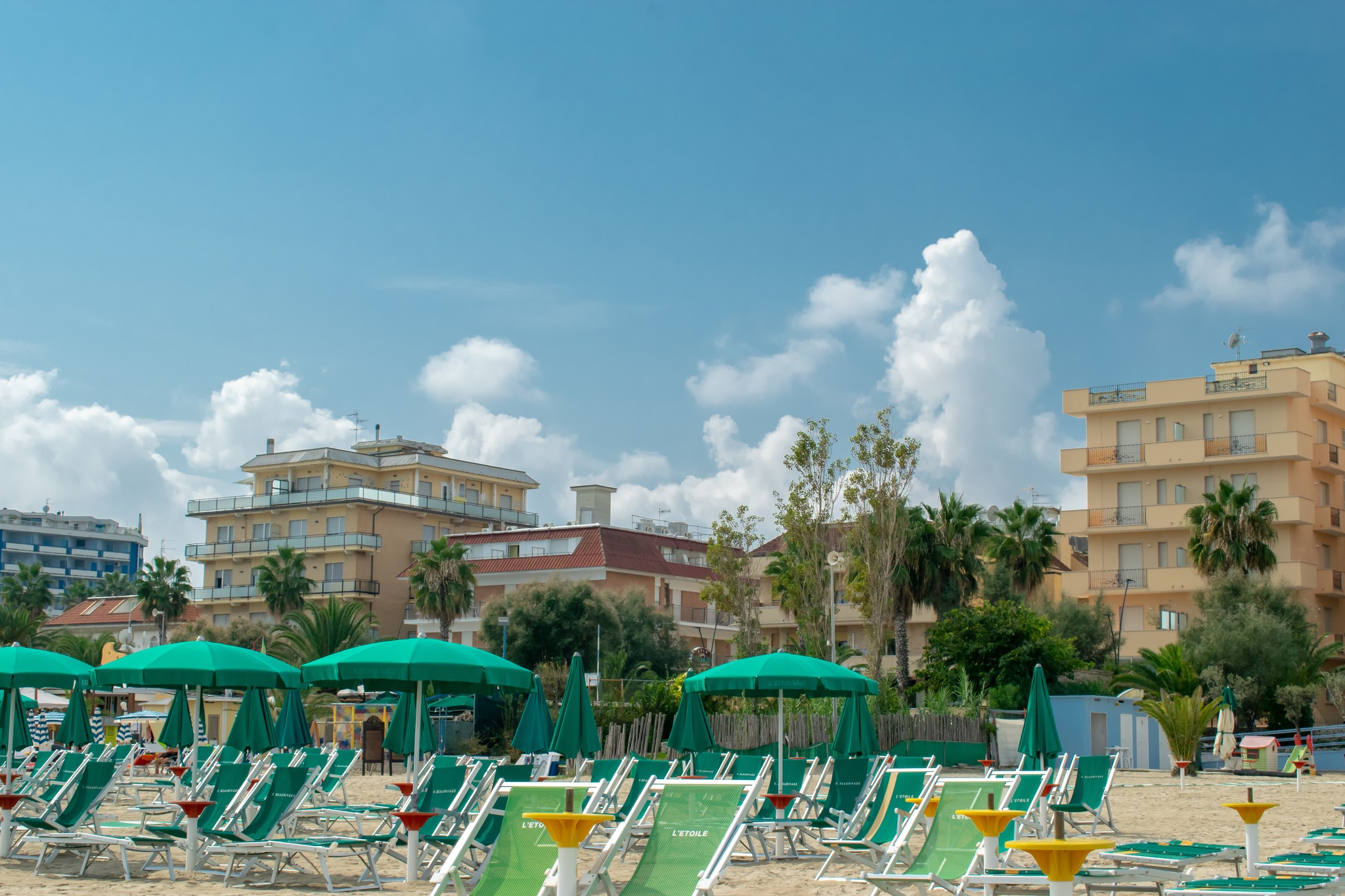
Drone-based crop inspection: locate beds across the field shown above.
[1162,876,1345,896]
[961,865,1195,896]
[1299,826,1345,853]
[1253,849,1345,879]
[1096,839,1249,885]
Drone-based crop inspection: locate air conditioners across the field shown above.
[645,526,653,532]
[543,522,554,527]
[685,532,694,539]
[482,528,492,532]
[114,561,120,567]
[506,526,518,531]
[662,529,670,535]
[566,521,576,526]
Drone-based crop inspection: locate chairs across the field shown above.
[969,766,1054,869]
[1018,749,1069,836]
[1,747,942,896]
[859,774,1022,896]
[1044,751,1121,840]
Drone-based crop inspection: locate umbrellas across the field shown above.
[27,711,66,745]
[338,686,474,721]
[89,635,302,801]
[682,651,879,795]
[114,710,168,752]
[302,634,538,812]
[0,642,113,794]
[0,689,38,709]
[19,686,70,710]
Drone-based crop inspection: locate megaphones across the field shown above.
[152,608,163,616]
[827,551,844,565]
